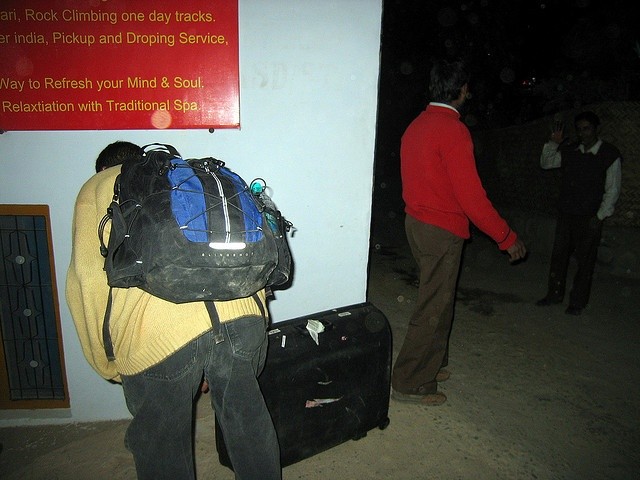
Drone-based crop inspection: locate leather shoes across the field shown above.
[395,389,448,406]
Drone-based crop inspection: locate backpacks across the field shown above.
[97,144,294,304]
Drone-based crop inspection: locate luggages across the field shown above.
[215,302,393,476]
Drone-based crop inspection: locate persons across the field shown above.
[536,110,623,315]
[64,140,295,479]
[391,53,528,406]
